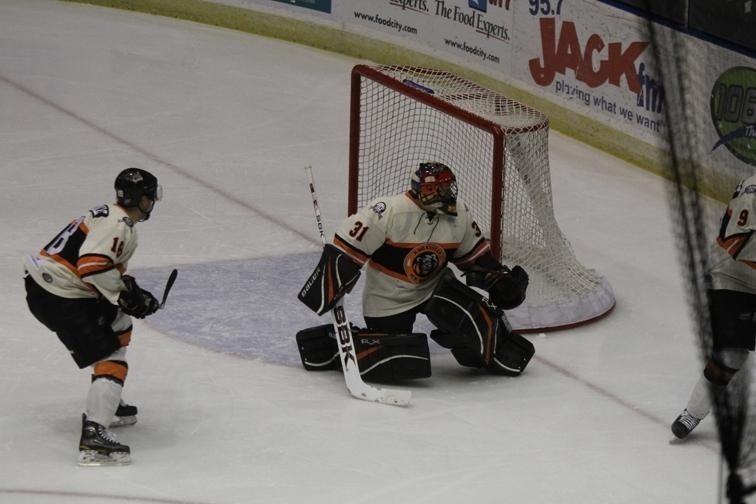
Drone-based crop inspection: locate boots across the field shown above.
[79,411,130,453]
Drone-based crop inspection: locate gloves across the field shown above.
[118,274,159,320]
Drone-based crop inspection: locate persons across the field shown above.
[295,160,535,382]
[669,168,754,439]
[21,167,161,459]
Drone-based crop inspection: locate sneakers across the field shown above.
[115,396,137,416]
[670,407,701,438]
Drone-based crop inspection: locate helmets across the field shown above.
[412,162,459,218]
[113,167,158,208]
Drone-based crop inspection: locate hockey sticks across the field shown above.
[304,164,412,407]
[158,269,177,309]
